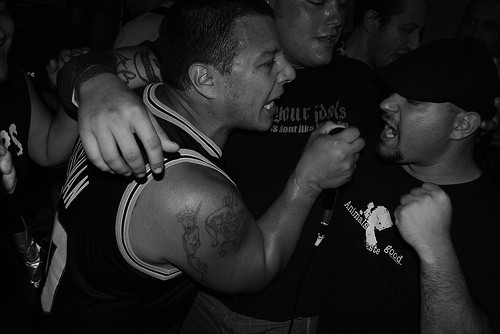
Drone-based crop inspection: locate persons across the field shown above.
[0,0,500,334]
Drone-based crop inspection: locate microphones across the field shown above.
[314,127,348,247]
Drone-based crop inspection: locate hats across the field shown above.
[388,36,498,121]
